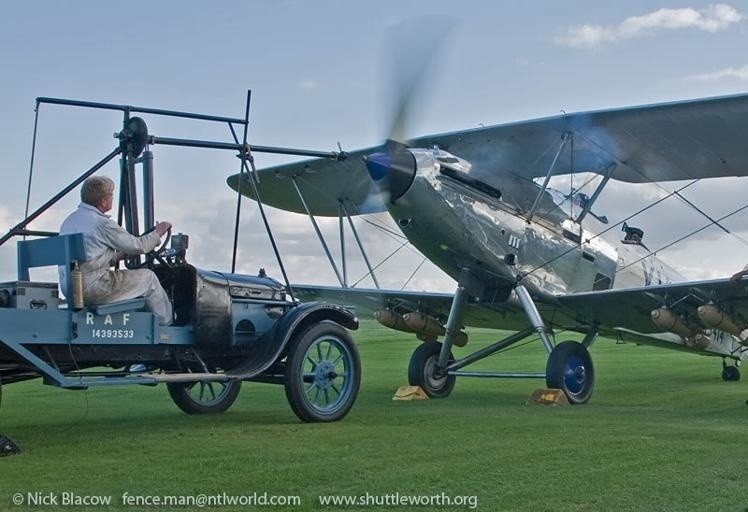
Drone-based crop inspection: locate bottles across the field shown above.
[68,257,85,309]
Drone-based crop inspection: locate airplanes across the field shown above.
[226,92,745,405]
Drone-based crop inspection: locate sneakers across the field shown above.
[170,310,190,327]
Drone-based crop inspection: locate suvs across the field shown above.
[0,86,363,456]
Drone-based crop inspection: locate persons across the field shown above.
[58,175,179,326]
